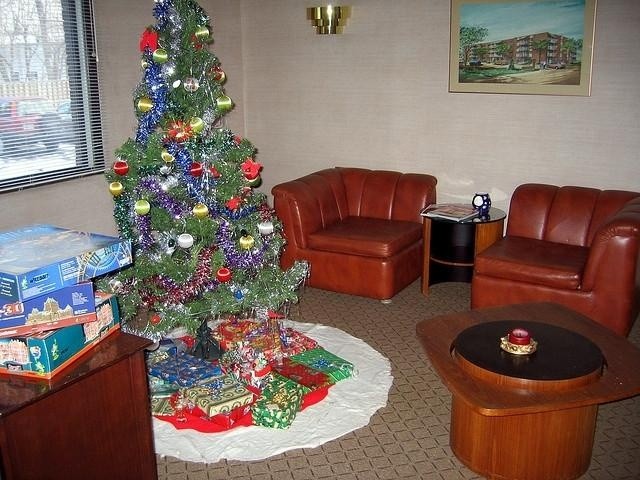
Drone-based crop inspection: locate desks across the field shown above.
[0,331,160,479]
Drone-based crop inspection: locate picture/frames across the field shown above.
[449,0,596,98]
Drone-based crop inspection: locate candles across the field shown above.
[508,326,531,345]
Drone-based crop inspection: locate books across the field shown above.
[420,205,479,222]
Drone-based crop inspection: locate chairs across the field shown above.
[270,167,437,305]
[469,182,639,341]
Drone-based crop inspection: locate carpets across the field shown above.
[151,320,394,464]
